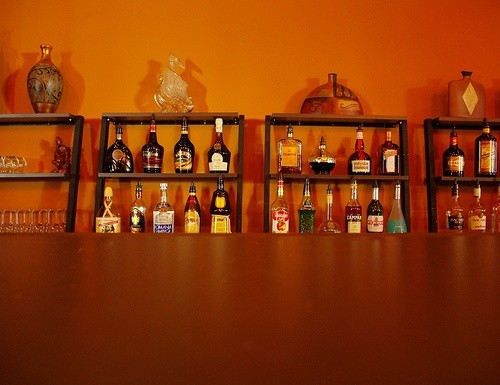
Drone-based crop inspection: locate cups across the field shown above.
[0,155,27,175]
[0,208,66,232]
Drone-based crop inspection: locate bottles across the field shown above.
[296,178,317,234]
[276,126,304,175]
[447,70,487,120]
[27,44,63,113]
[207,118,231,173]
[308,137,336,175]
[467,179,487,232]
[491,185,500,233]
[184,186,202,234]
[94,186,122,234]
[445,178,465,233]
[344,180,363,233]
[474,118,498,177]
[379,130,401,175]
[300,73,365,116]
[442,132,466,178]
[128,181,147,233]
[271,178,291,234]
[104,124,134,173]
[317,185,342,233]
[152,183,175,234]
[386,179,408,234]
[209,178,231,234]
[347,129,373,175]
[141,119,164,174]
[365,180,384,233]
[174,119,195,174]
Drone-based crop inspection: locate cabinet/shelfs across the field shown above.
[0,116,85,233]
[263,113,412,233]
[424,116,500,233]
[92,112,246,232]
